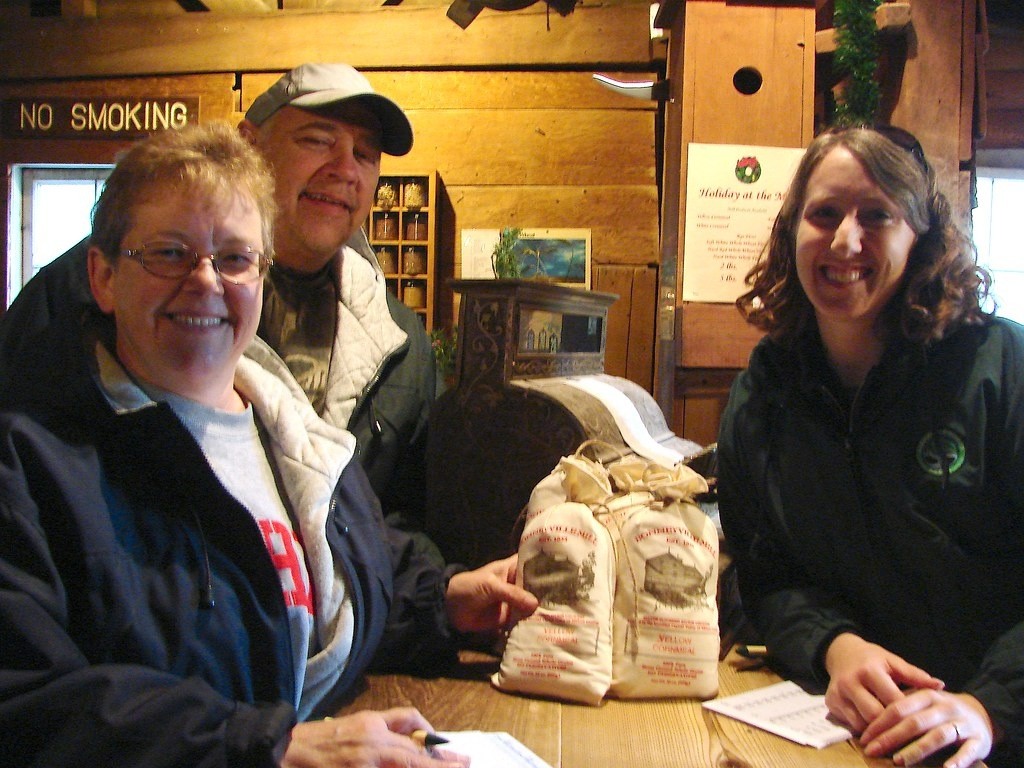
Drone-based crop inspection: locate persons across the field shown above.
[717,125,1024,768]
[0,131,541,768]
[1,64,465,570]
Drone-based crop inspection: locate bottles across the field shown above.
[403,246,426,274]
[374,212,397,240]
[376,178,399,207]
[403,280,426,309]
[404,213,428,240]
[404,177,426,207]
[374,246,397,274]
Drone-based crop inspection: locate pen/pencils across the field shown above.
[736,644,768,658]
[323,715,450,749]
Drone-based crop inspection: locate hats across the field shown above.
[244,63,415,157]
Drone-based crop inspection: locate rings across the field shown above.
[951,720,963,742]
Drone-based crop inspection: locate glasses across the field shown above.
[118,239,274,286]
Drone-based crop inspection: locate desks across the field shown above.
[320,626,948,768]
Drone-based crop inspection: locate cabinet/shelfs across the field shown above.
[444,279,620,385]
[359,169,441,338]
